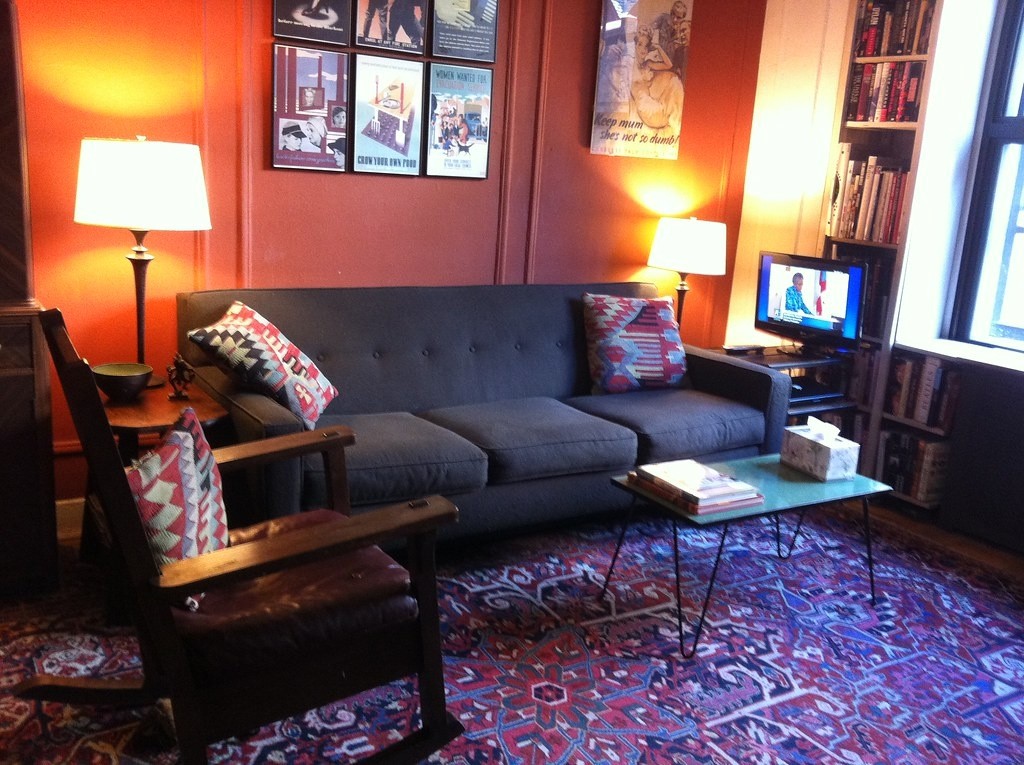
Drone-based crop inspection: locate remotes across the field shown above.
[723,344,766,354]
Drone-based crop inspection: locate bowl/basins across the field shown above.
[92,362,153,402]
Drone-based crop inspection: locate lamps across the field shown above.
[73,137,213,389]
[647,217,727,332]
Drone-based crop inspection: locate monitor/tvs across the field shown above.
[754,251,869,359]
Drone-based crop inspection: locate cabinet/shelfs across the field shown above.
[717,345,857,441]
[0,1,58,603]
[789,0,1023,547]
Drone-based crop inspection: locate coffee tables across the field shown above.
[595,454,894,657]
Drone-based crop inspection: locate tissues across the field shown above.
[779,416,861,482]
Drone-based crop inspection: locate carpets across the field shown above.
[0,505,1024,764]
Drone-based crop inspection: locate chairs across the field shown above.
[38,309,466,765]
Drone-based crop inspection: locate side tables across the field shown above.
[78,374,227,564]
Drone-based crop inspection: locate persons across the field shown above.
[304,88,316,106]
[282,121,307,152]
[333,107,346,128]
[786,273,812,314]
[363,0,425,45]
[306,117,328,148]
[328,138,345,169]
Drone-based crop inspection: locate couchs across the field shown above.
[176,282,792,550]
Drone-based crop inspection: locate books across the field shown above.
[627,459,765,515]
[838,0,936,244]
[822,350,960,502]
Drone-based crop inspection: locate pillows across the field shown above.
[581,293,693,395]
[185,301,339,431]
[121,405,228,613]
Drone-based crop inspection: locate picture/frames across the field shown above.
[270,0,499,180]
